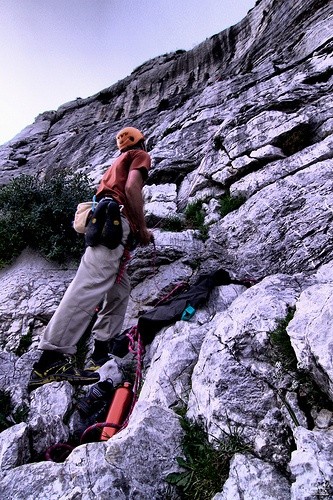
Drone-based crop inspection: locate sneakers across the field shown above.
[83,358,112,370]
[28,356,100,386]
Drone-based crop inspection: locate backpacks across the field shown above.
[137,269,232,346]
[63,378,117,445]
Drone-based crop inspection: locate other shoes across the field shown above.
[86,201,123,249]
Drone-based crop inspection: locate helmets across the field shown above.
[116,127,145,151]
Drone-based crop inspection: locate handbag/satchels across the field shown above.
[73,202,99,233]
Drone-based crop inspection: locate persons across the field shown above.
[27,126,153,385]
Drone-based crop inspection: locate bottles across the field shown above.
[100,381,133,440]
